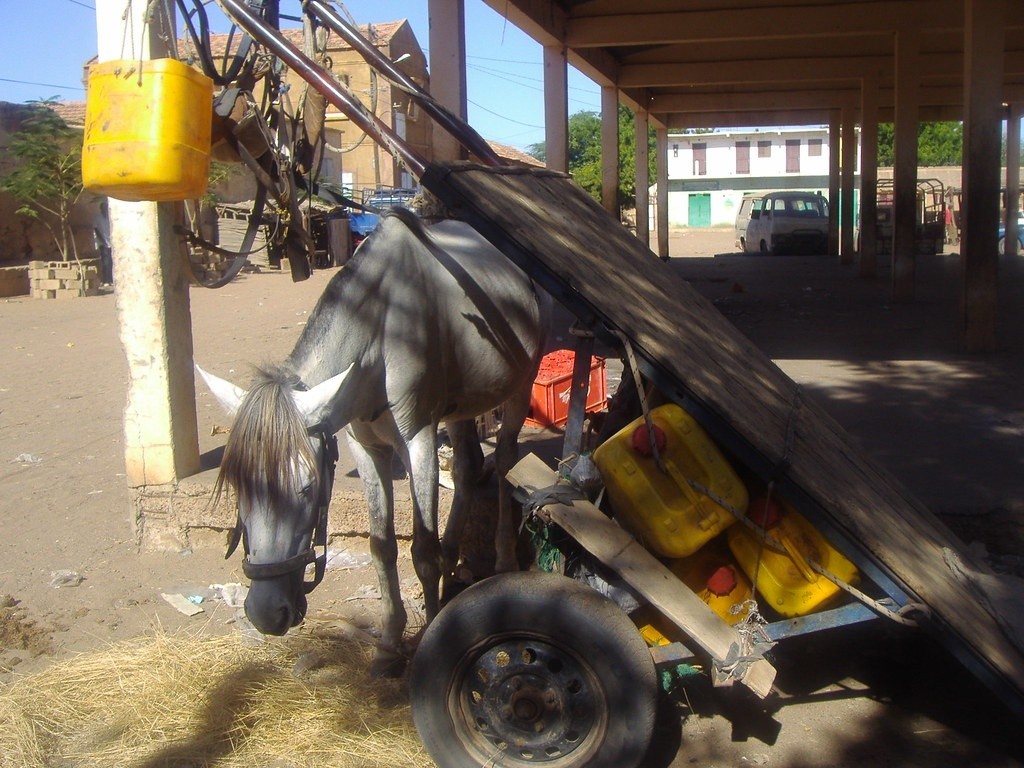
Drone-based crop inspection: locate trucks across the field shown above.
[856,178,945,255]
[338,186,417,249]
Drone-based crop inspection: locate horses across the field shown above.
[195,203,545,711]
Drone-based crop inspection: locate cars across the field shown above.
[998,210,1024,255]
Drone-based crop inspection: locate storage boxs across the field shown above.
[522,349,607,427]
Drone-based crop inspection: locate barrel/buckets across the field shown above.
[669,549,758,627]
[726,495,860,618]
[81,58,215,202]
[592,402,750,560]
[627,603,692,647]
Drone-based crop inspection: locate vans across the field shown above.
[734,190,829,254]
